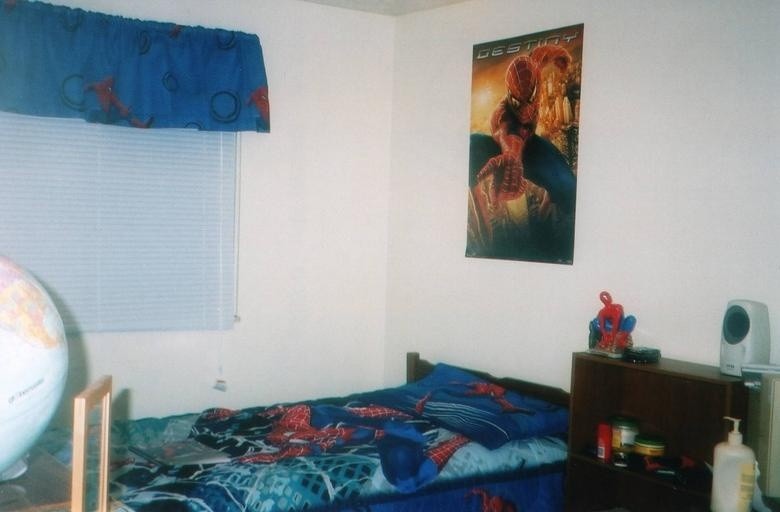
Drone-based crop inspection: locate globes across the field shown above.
[0,254,70,480]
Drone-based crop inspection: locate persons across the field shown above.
[589,290,635,359]
[468,44,576,234]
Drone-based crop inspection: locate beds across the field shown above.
[42,352,616,511]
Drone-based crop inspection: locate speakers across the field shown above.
[719,298,771,377]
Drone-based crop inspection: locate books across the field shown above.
[128,437,232,470]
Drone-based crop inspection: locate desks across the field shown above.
[0,443,97,511]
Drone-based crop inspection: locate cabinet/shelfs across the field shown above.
[565,346,758,511]
